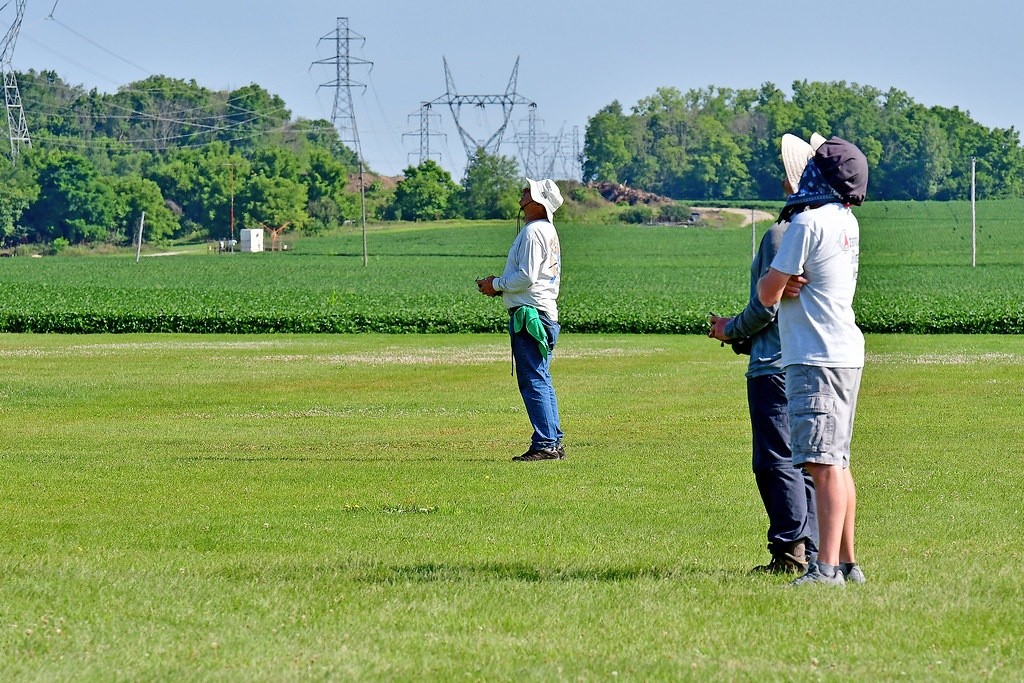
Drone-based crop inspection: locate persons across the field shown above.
[475,177,566,460]
[710,132,867,589]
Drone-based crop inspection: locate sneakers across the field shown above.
[785,563,846,590]
[556,443,566,459]
[512,445,559,462]
[843,565,867,583]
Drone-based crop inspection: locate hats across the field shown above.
[782,133,817,194]
[526,177,564,223]
[811,132,827,151]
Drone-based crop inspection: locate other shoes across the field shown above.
[806,549,818,568]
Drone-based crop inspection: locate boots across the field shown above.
[753,539,808,575]
